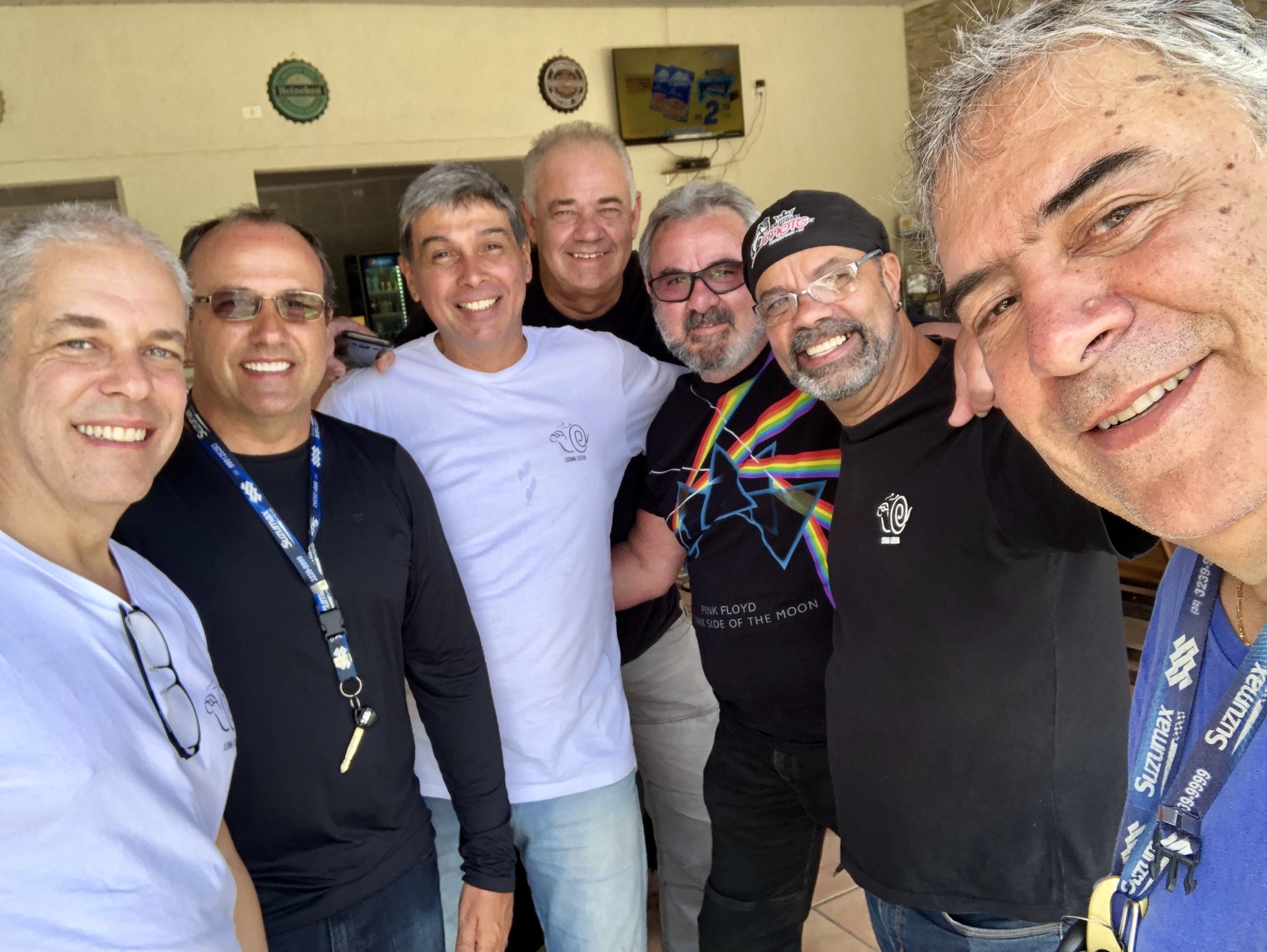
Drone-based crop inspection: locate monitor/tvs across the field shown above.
[611,43,745,146]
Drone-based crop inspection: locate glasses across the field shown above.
[751,248,886,327]
[647,260,745,303]
[118,602,201,761]
[193,287,330,322]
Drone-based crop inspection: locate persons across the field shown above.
[325,124,720,952]
[110,201,517,952]
[741,191,1157,952]
[316,164,691,952]
[903,0,1267,952]
[611,179,998,952]
[0,202,265,952]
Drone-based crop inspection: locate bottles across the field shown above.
[370,300,400,313]
[375,320,401,340]
[366,275,392,291]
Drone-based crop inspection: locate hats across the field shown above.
[742,190,892,303]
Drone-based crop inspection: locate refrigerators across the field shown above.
[344,251,423,348]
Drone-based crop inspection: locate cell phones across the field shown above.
[332,330,393,369]
